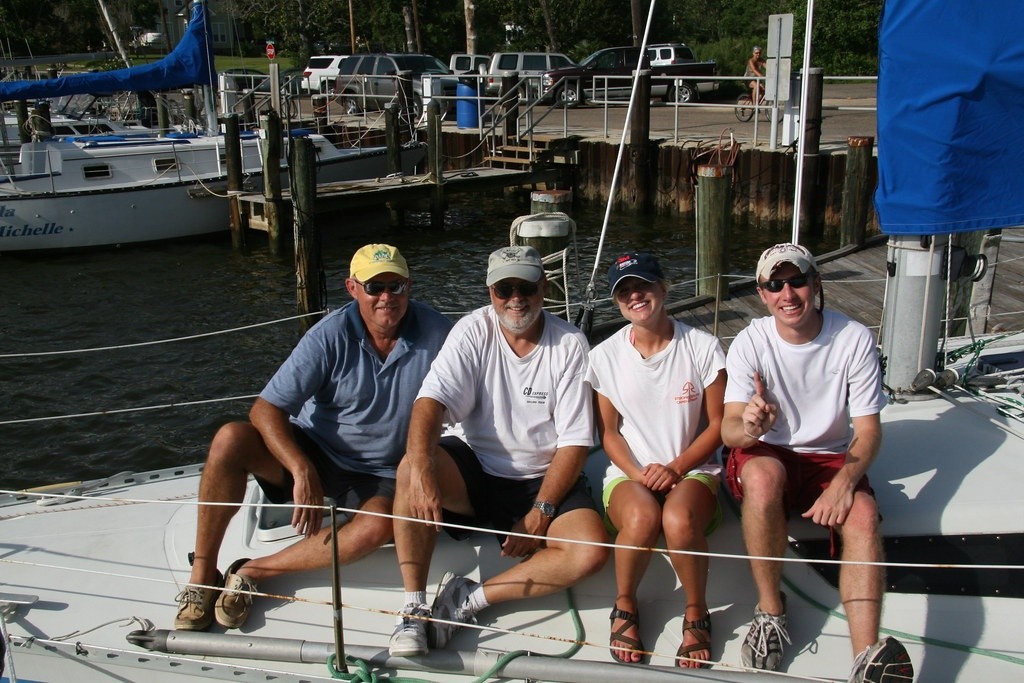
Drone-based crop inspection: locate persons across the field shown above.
[720,243,914,683]
[389,246,610,657]
[744,46,766,113]
[173,244,454,631]
[583,252,728,669]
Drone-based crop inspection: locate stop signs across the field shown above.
[265,43,275,61]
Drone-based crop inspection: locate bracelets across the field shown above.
[744,428,777,438]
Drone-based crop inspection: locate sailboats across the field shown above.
[1,0,1024,682]
[0,0,431,265]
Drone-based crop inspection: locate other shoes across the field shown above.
[753,109,761,113]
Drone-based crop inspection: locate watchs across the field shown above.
[534,501,554,517]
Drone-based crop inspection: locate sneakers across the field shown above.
[174,569,224,630]
[740,591,792,671]
[388,602,431,656]
[427,570,478,650]
[847,635,915,683]
[215,557,258,628]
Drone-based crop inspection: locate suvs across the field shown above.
[334,53,454,119]
[647,43,694,65]
[478,52,578,106]
[301,54,349,100]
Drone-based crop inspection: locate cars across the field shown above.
[259,68,307,99]
[220,68,267,94]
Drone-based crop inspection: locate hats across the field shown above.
[349,244,409,282]
[608,251,666,297]
[755,243,819,284]
[486,246,545,287]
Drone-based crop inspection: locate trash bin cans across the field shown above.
[456,81,485,128]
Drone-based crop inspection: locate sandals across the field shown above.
[675,609,713,668]
[609,603,646,665]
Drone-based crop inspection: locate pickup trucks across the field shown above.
[449,53,492,76]
[541,46,717,109]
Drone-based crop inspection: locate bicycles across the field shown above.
[735,87,784,124]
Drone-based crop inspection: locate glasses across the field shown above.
[760,270,817,293]
[754,51,761,54]
[489,276,546,300]
[353,278,409,295]
